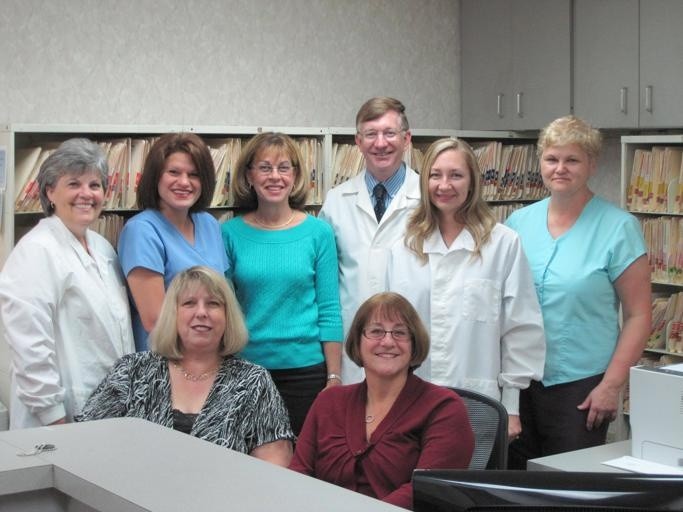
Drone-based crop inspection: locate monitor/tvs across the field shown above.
[411,468,683,511]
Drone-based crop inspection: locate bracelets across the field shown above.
[326,375,342,380]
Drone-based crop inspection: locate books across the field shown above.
[622,145,683,414]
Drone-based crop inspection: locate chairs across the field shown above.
[447,385,509,471]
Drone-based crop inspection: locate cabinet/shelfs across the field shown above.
[460,0,573,131]
[1,121,552,433]
[572,0,683,129]
[617,135,683,440]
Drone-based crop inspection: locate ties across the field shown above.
[373,184,387,224]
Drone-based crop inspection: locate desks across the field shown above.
[527,440,682,472]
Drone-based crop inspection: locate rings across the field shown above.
[515,436,519,440]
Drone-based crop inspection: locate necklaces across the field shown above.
[254,210,297,228]
[172,360,219,380]
[363,413,382,423]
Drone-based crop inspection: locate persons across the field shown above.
[218,132,343,433]
[293,292,473,509]
[73,264,294,469]
[316,97,422,386]
[0,137,136,431]
[377,136,545,446]
[117,134,232,351]
[500,116,653,473]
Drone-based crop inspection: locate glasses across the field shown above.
[249,160,292,175]
[363,325,412,341]
[358,127,402,139]
